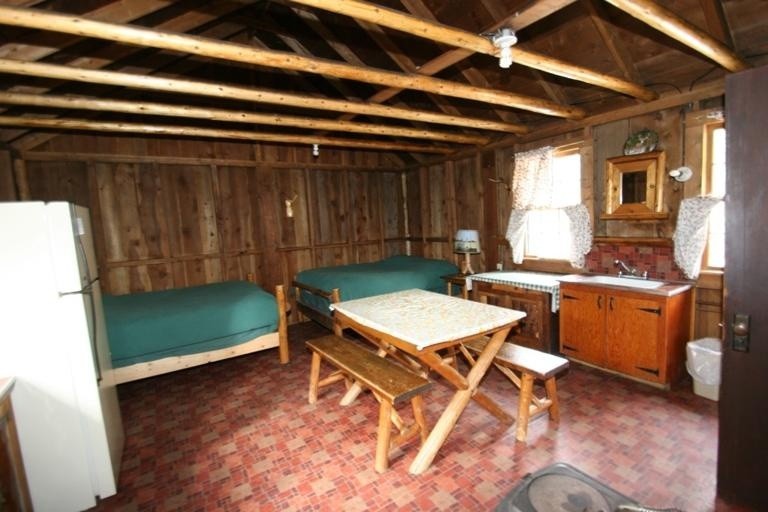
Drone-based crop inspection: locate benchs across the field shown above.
[303,334,433,473]
[463,333,571,441]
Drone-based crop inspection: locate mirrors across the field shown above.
[601,150,666,222]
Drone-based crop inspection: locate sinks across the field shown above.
[583,275,664,290]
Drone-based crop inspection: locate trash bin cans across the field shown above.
[686,337,724,402]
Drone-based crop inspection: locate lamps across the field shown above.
[667,166,693,182]
[312,143,320,156]
[451,230,481,275]
[492,1,519,70]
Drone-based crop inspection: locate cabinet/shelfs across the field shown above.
[471,269,587,355]
[554,273,693,392]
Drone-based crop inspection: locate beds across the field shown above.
[291,253,461,337]
[99,272,289,388]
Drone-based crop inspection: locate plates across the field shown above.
[623,130,657,156]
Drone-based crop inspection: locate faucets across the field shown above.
[614,259,637,275]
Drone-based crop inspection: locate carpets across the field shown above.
[486,463,677,512]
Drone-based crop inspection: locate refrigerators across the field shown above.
[1,200,127,512]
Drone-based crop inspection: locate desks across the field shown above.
[1,375,37,511]
[331,286,528,475]
[440,273,476,300]
